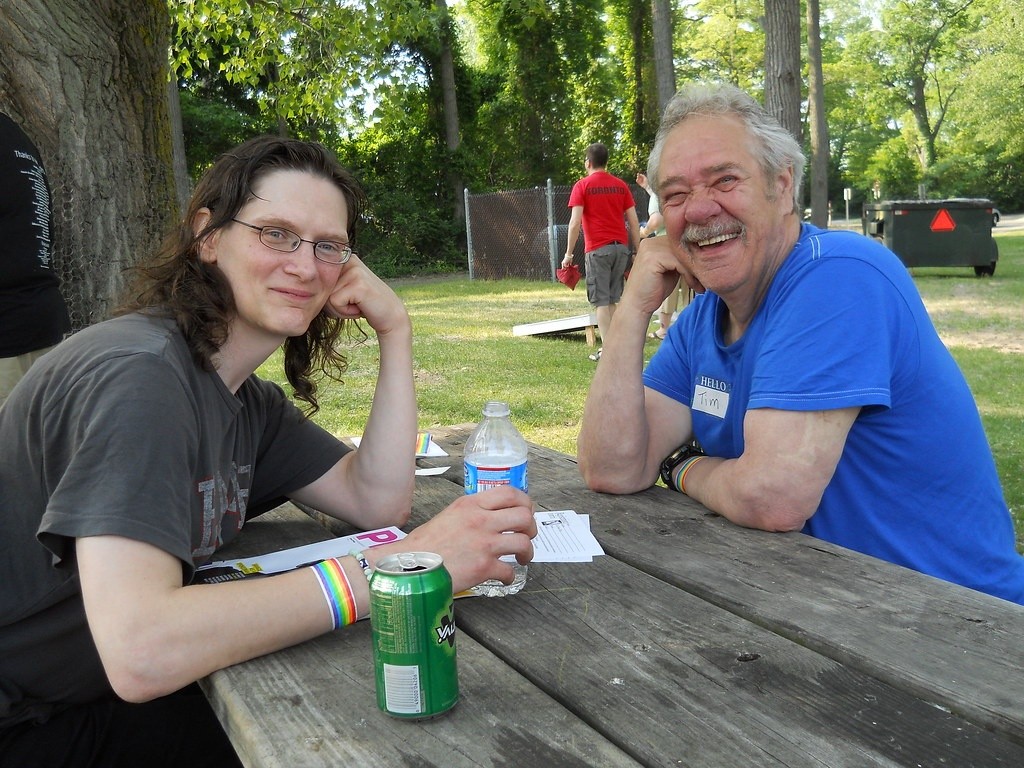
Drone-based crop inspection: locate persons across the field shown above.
[636,173,696,340]
[0,112,73,410]
[561,143,640,361]
[577,80,1024,607]
[0,134,538,768]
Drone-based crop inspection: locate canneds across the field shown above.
[367,551,459,723]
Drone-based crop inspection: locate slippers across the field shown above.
[648,332,664,340]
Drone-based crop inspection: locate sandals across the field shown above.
[590,352,601,362]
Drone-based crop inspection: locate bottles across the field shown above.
[463,400,527,597]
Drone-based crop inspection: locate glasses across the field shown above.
[226,217,351,264]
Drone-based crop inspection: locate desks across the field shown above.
[197,422,1024,768]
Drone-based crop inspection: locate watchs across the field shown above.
[659,444,708,493]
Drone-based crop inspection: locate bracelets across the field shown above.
[675,456,709,494]
[564,253,574,259]
[310,557,358,632]
[349,549,373,581]
[631,252,637,255]
[643,229,648,237]
